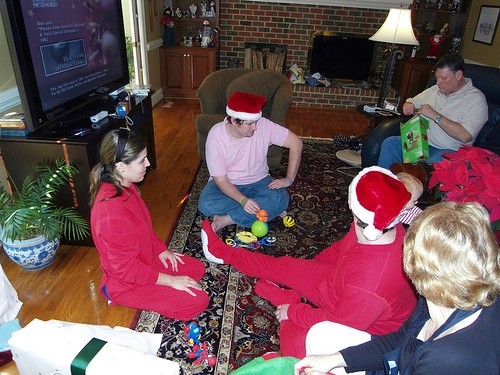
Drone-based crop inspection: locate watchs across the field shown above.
[434,114,441,124]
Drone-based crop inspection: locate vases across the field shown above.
[165,30,174,44]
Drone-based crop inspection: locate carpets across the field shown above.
[131,137,361,375]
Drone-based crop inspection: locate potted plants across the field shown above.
[0,158,90,271]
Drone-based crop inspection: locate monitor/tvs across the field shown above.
[0,0,130,132]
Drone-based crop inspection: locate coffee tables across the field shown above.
[356,104,401,132]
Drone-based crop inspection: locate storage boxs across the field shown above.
[7,319,179,375]
[400,115,429,163]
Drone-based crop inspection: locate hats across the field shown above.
[225,91,267,121]
[347,166,411,241]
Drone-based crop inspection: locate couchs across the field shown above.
[361,63,500,169]
[194,68,294,170]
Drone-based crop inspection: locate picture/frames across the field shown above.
[472,5,500,46]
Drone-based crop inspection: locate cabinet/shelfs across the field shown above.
[159,0,219,99]
[392,0,472,116]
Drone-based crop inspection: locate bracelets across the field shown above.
[285,177,294,184]
[240,197,247,206]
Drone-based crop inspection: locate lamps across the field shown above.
[369,7,419,109]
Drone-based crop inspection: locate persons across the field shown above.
[89,130,209,322]
[161,6,175,45]
[202,166,418,359]
[198,92,303,233]
[292,201,500,375]
[378,53,490,170]
[199,19,214,46]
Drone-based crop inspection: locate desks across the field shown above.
[0,89,156,247]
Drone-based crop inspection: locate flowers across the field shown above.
[427,144,500,232]
[161,15,176,32]
